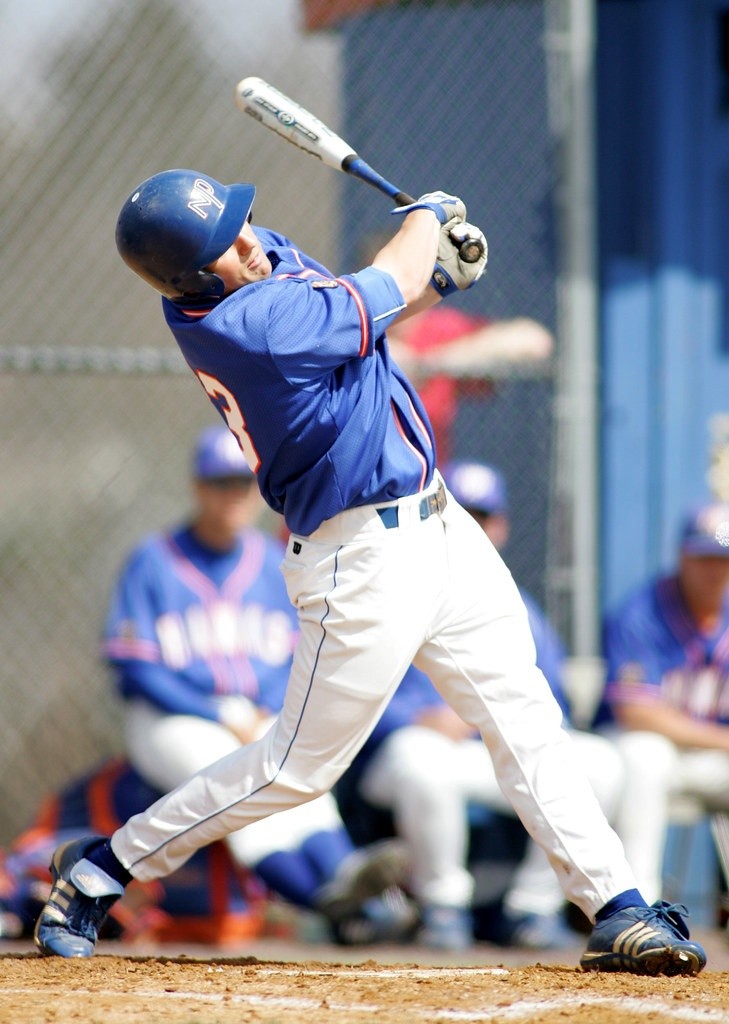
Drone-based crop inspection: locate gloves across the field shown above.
[391,191,467,225]
[430,217,488,298]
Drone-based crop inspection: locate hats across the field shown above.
[683,503,729,556]
[193,425,256,479]
[449,462,508,513]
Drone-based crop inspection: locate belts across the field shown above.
[375,485,447,528]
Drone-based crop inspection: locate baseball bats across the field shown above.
[236,75,489,267]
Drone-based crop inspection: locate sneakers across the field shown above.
[580,899,706,974]
[34,835,125,959]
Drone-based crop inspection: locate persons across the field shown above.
[33,170,709,983]
[590,500,728,911]
[331,459,627,959]
[99,424,418,948]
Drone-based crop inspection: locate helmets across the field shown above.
[115,169,257,305]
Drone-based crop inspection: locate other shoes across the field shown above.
[502,914,588,954]
[336,888,420,945]
[317,840,411,922]
[415,907,471,951]
